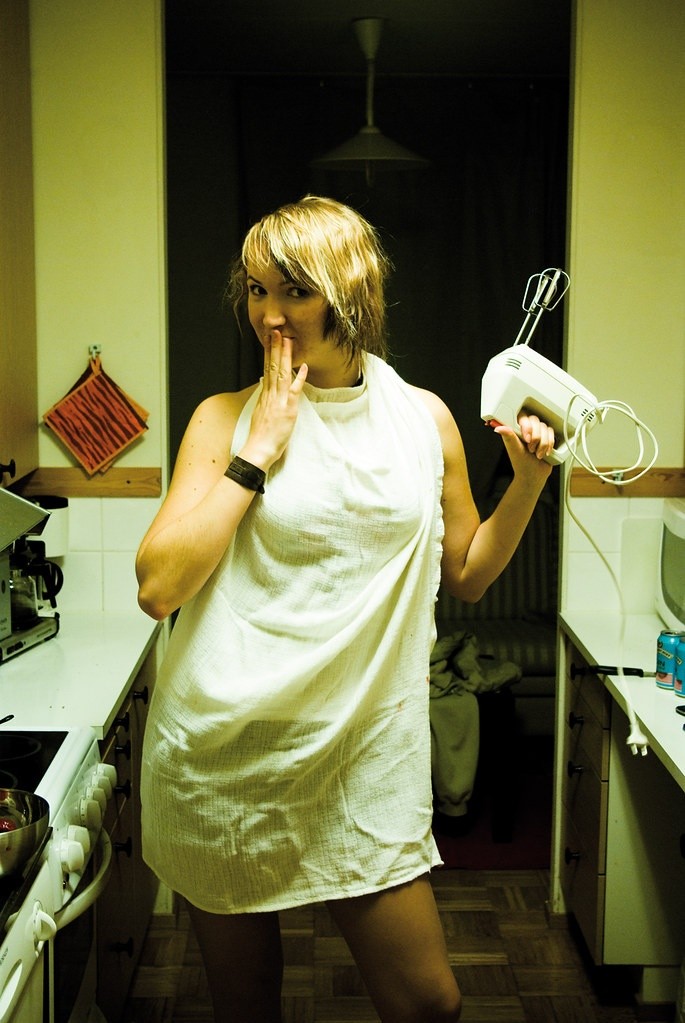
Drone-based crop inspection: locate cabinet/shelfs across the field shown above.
[96,629,169,1023]
[558,642,685,1007]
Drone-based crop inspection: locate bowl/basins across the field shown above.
[0,788,49,881]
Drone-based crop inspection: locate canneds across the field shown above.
[656,629,685,698]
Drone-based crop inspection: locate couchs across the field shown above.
[429,497,560,736]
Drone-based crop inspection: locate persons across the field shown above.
[133,196,555,1022]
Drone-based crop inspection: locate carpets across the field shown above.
[117,867,639,1023]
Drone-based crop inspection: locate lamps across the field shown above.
[308,14,435,188]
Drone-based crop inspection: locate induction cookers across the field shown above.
[0,723,121,1022]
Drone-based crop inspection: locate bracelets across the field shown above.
[225,456,267,494]
